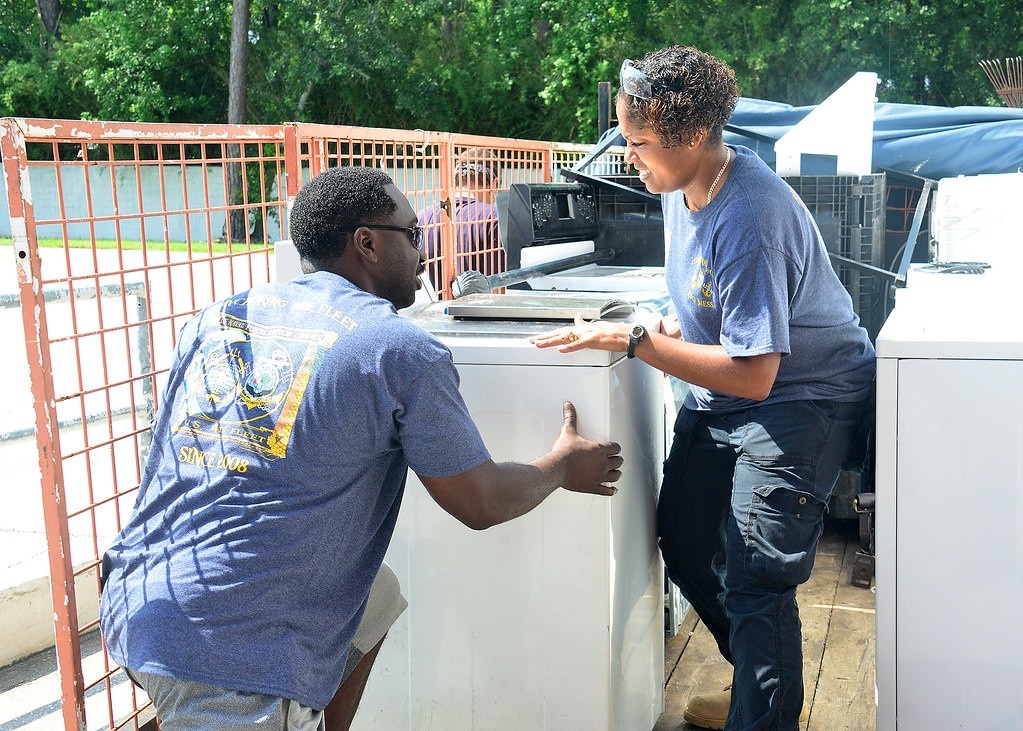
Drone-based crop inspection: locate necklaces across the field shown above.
[685,146,730,208]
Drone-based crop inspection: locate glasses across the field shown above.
[352,223,423,251]
[620,59,680,101]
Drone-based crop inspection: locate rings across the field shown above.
[568,332,578,342]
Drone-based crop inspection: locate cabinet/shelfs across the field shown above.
[347,262,1023,731]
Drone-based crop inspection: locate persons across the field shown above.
[528,46,876,731]
[100,167,624,731]
[417,148,501,300]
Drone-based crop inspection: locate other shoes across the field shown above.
[684,683,732,729]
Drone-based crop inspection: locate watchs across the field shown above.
[627,325,646,359]
